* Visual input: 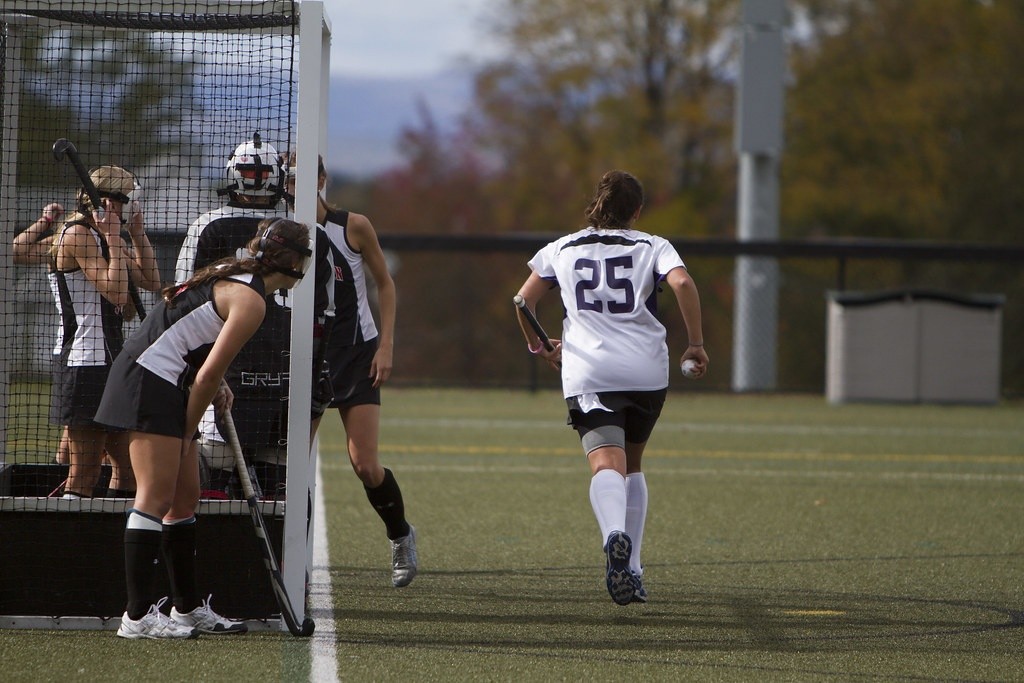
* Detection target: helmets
[226,139,284,198]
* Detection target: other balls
[680,359,701,379]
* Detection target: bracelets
[41,215,51,227]
[688,341,704,347]
[527,341,543,354]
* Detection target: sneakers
[116,596,200,640]
[607,530,647,606]
[170,594,249,634]
[390,525,417,586]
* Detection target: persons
[513,172,709,607]
[53,166,161,501]
[172,132,336,544]
[11,184,139,462]
[281,151,419,585]
[92,217,312,637]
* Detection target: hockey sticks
[511,292,564,369]
[50,135,148,320]
[215,382,317,638]
